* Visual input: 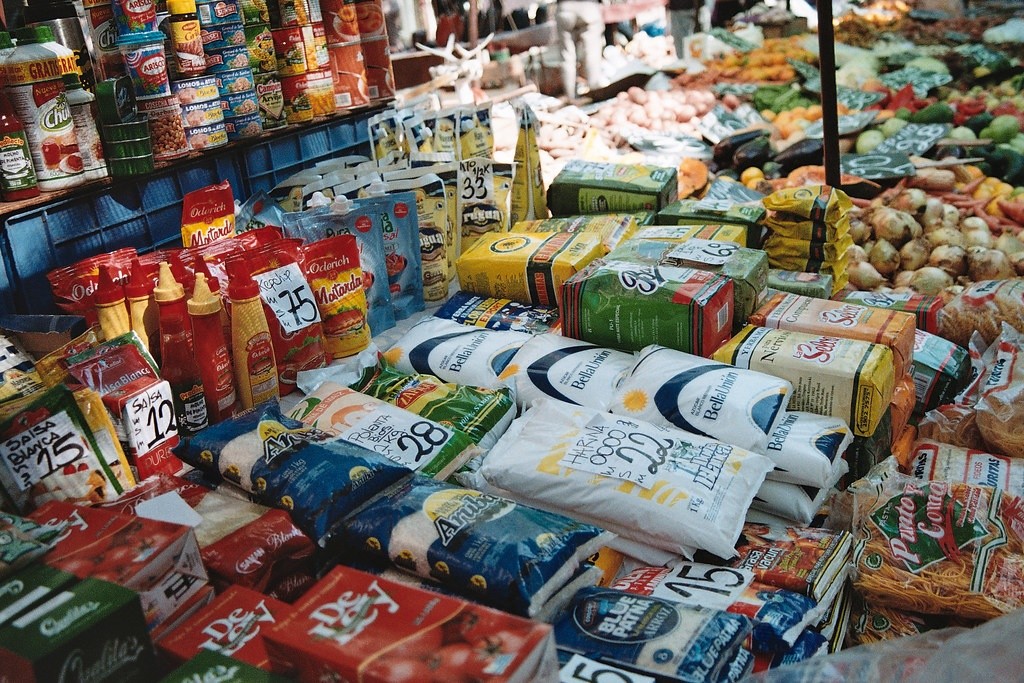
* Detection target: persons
[553,0,765,99]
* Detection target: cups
[111,0,159,34]
[115,30,171,101]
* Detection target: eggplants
[702,129,825,181]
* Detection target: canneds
[163,0,396,154]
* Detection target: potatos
[588,86,739,140]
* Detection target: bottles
[227,259,279,410]
[33,26,82,87]
[92,264,130,342]
[62,73,109,180]
[188,255,232,366]
[0,97,40,203]
[0,31,16,95]
[123,258,160,359]
[154,262,199,386]
[169,253,195,299]
[6,27,87,192]
[186,272,237,425]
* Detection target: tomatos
[375,608,531,683]
[52,519,179,586]
